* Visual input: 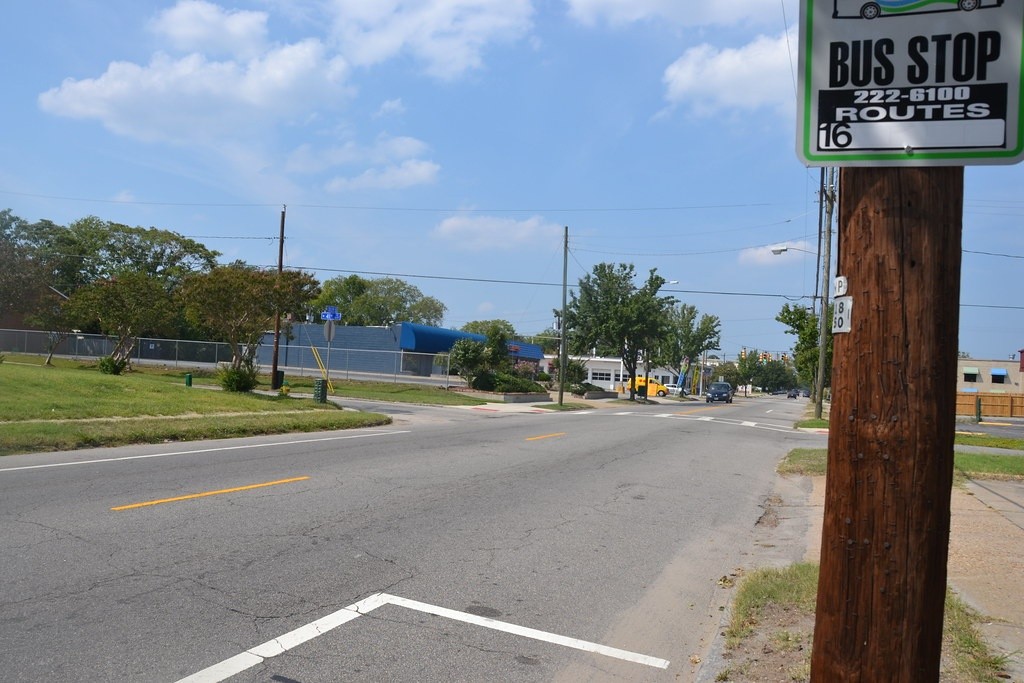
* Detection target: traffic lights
[759,352,772,363]
[741,349,747,360]
[781,353,788,363]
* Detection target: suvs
[664,384,690,396]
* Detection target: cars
[787,389,811,399]
[706,382,735,403]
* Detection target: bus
[627,377,670,397]
